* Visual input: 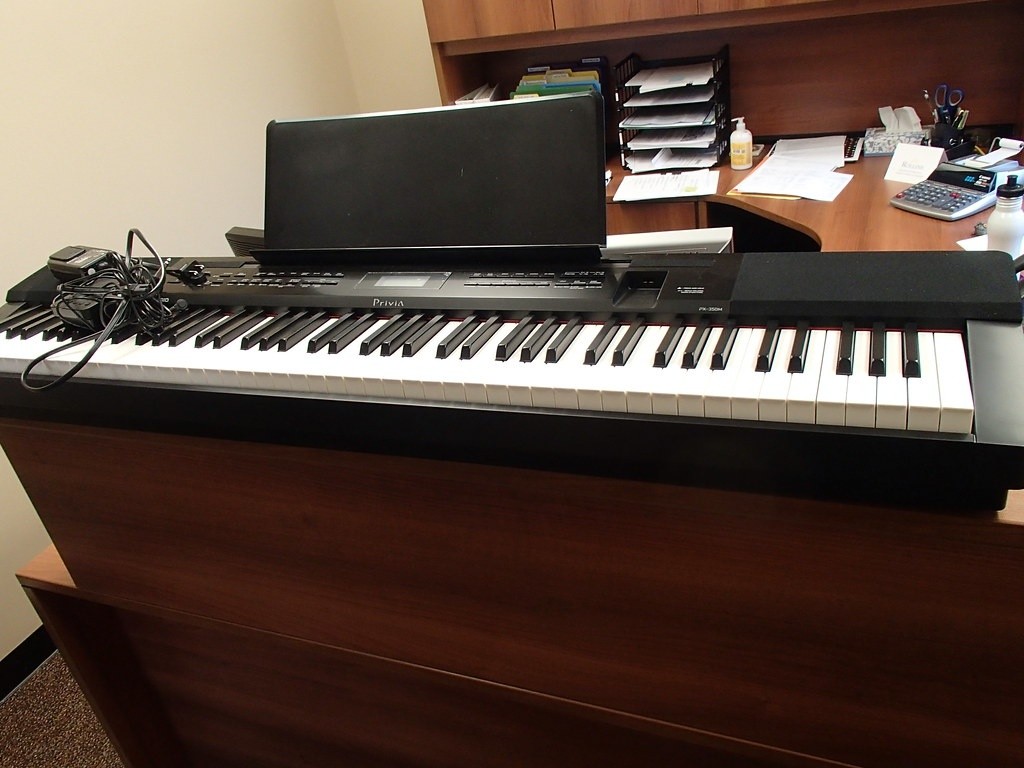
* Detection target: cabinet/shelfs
[599,196,708,230]
[424,1,1024,193]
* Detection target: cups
[937,121,965,150]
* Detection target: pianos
[0,243,1024,513]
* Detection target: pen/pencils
[930,107,969,129]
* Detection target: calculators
[890,162,998,222]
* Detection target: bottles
[730,117,753,170]
[987,175,1024,259]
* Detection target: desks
[606,148,1024,252]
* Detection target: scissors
[933,83,965,122]
